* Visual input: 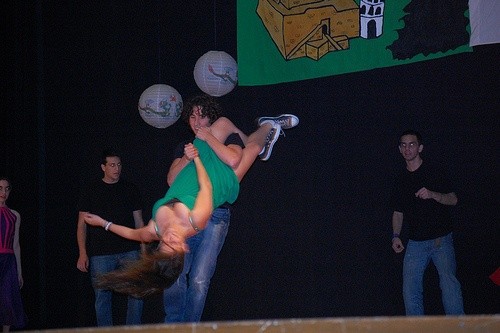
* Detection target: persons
[162,95,245,323]
[84,114,299,299]
[76,152,148,327]
[0,177,27,333]
[392,130,466,316]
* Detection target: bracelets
[105,221,112,231]
[392,234,399,241]
[439,193,442,203]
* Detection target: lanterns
[193,52,238,97]
[138,84,183,128]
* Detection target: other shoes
[257,123,281,161]
[257,113,300,129]
